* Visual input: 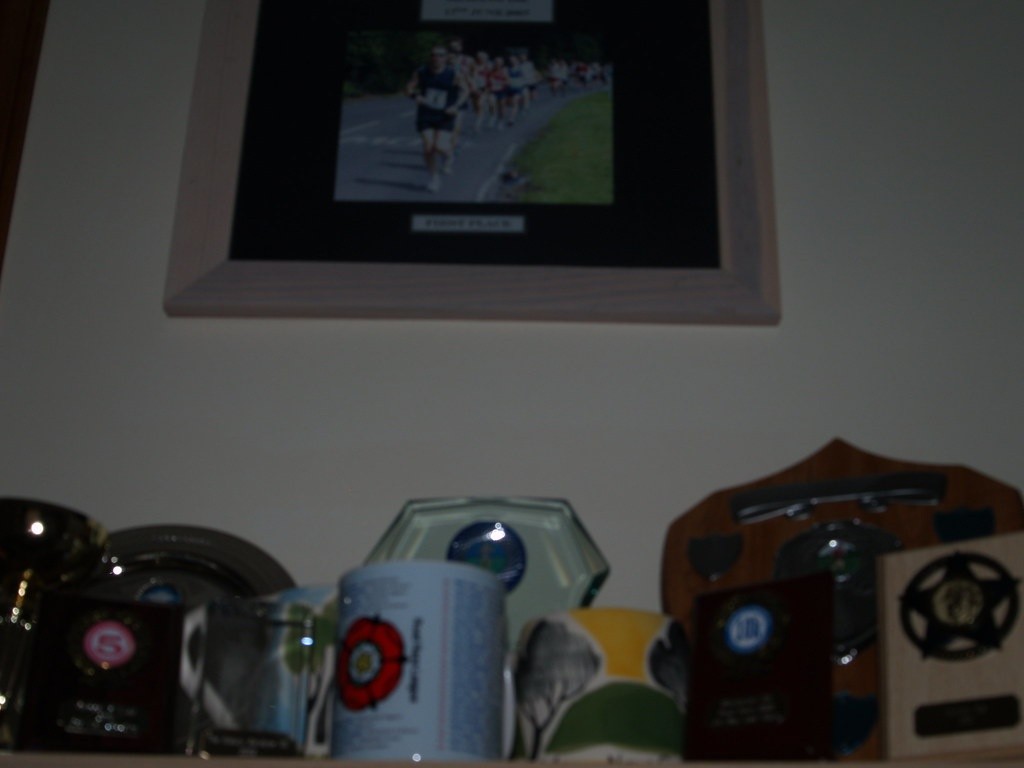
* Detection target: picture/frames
[163,1,782,326]
